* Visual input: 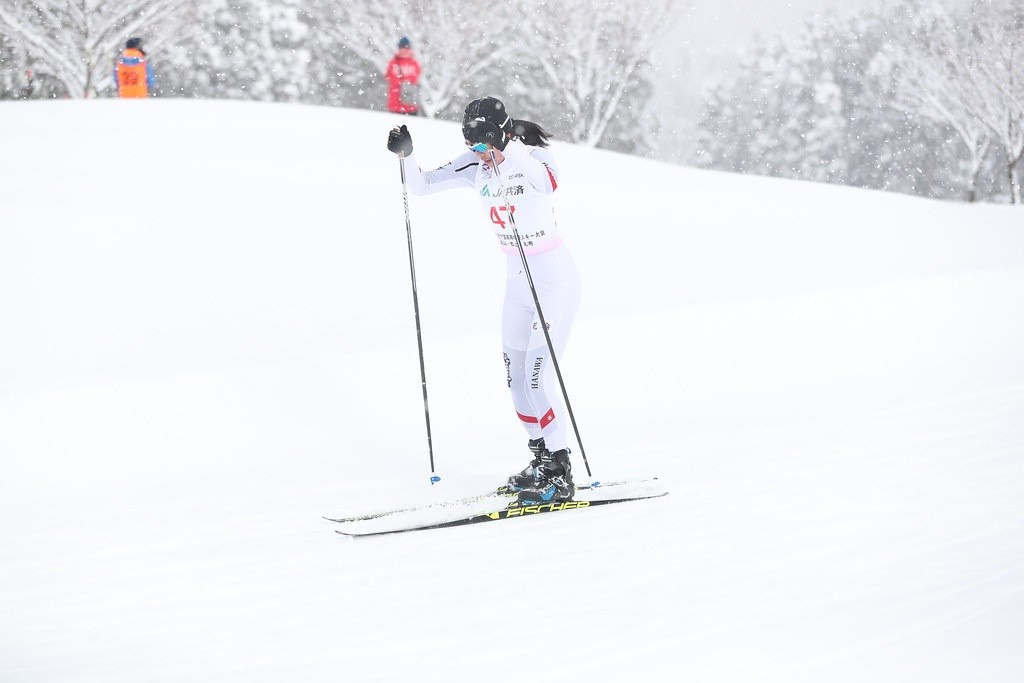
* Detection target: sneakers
[508,437,545,488]
[518,448,575,502]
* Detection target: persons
[384,37,422,115]
[113,37,155,98]
[387,96,582,505]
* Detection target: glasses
[464,141,488,152]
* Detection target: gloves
[388,125,413,157]
[462,117,509,151]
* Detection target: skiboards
[321,477,669,539]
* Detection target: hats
[126,38,140,47]
[398,37,409,48]
[461,95,511,135]
